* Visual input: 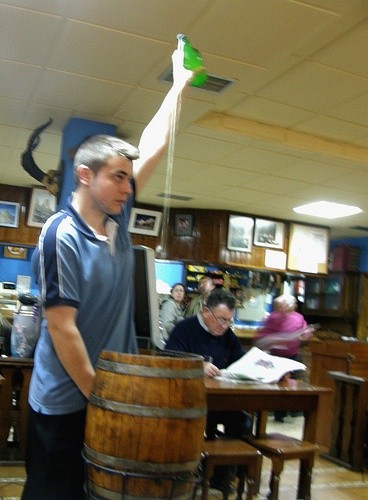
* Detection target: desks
[319,368,368,475]
[202,371,336,500]
[0,356,35,467]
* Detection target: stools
[245,433,330,500]
[195,439,263,500]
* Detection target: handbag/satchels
[10,314,41,358]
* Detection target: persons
[20,48,206,500]
[184,276,215,318]
[160,283,185,343]
[164,289,245,495]
[251,293,313,423]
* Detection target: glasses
[203,305,234,328]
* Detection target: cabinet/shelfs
[183,264,357,338]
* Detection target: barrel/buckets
[82,347,208,500]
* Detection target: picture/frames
[174,212,194,236]
[254,217,285,249]
[226,214,254,252]
[0,199,21,228]
[128,206,163,237]
[26,185,59,228]
